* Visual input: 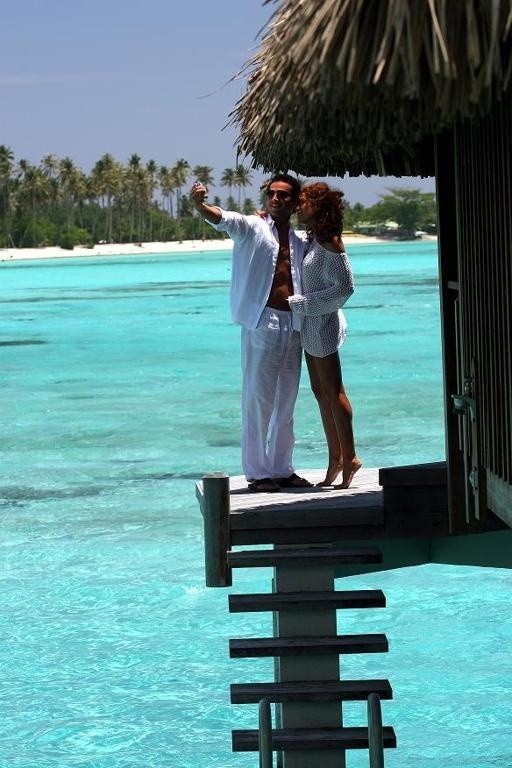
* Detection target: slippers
[248,478,280,492]
[273,473,314,488]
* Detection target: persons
[189,173,314,493]
[254,181,362,490]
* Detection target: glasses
[266,190,291,199]
